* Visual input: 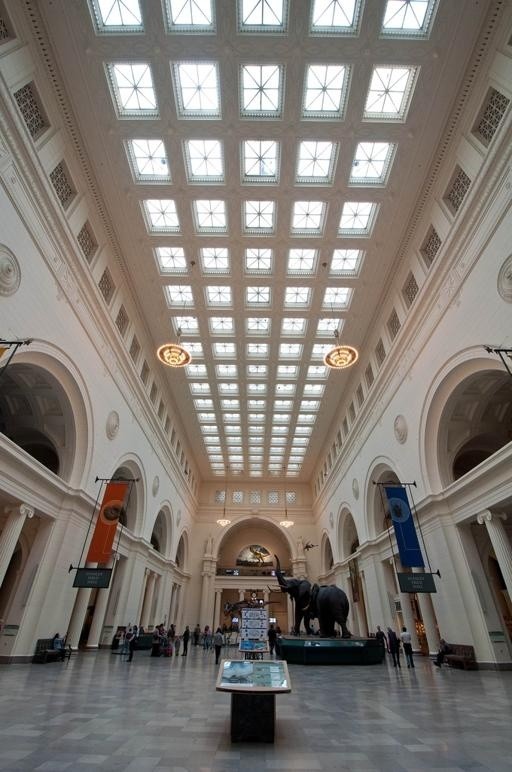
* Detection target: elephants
[266,554,351,638]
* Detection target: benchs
[442,643,474,670]
[32,637,66,664]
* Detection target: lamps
[215,464,296,529]
[321,262,359,371]
[155,260,196,369]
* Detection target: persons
[201,625,209,649]
[431,639,451,667]
[193,623,201,647]
[398,627,415,669]
[181,625,190,656]
[375,625,388,662]
[213,627,224,664]
[174,636,180,656]
[266,624,277,656]
[274,626,281,634]
[52,632,67,662]
[385,626,401,668]
[109,620,176,662]
[219,622,239,633]
